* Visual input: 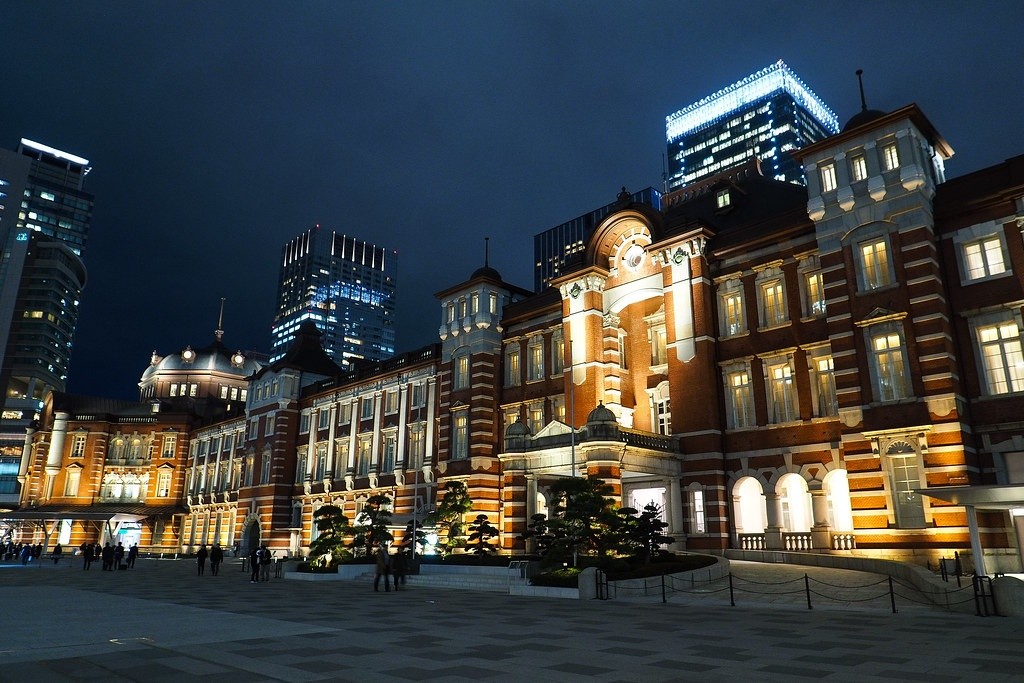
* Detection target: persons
[373,545,407,592]
[127,542,138,568]
[80,542,102,570]
[210,543,223,576]
[0,542,42,566]
[102,542,124,571]
[53,544,62,564]
[233,542,239,557]
[250,545,271,584]
[196,544,208,577]
